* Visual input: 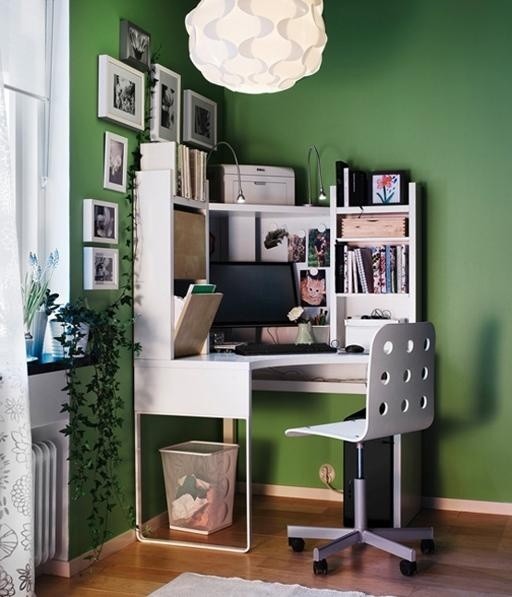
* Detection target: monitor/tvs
[208,261,302,344]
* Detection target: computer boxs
[343,407,393,528]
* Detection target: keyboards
[236,342,337,356]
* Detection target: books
[139,141,206,200]
[341,243,409,294]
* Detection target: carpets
[146,570,402,597]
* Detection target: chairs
[284,320,438,579]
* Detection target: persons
[314,233,325,266]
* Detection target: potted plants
[28,288,60,356]
[23,278,50,363]
[48,42,161,577]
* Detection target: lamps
[206,139,246,204]
[302,144,328,207]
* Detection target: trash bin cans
[158,441,240,535]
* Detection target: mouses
[345,344,365,354]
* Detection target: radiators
[28,438,57,567]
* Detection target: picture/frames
[367,167,411,204]
[82,16,219,293]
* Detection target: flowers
[23,247,60,335]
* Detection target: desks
[133,167,424,554]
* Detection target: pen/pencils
[309,309,328,325]
